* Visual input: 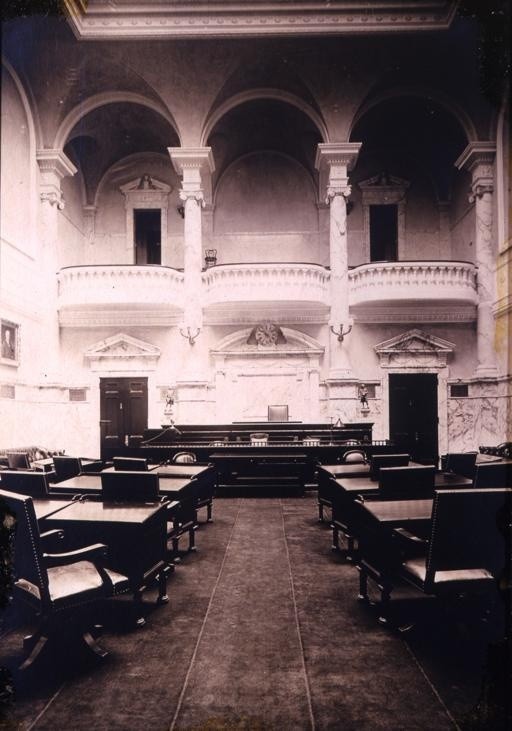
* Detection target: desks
[1,447,217,625]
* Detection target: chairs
[208,432,388,446]
[316,442,512,637]
[1,489,130,673]
